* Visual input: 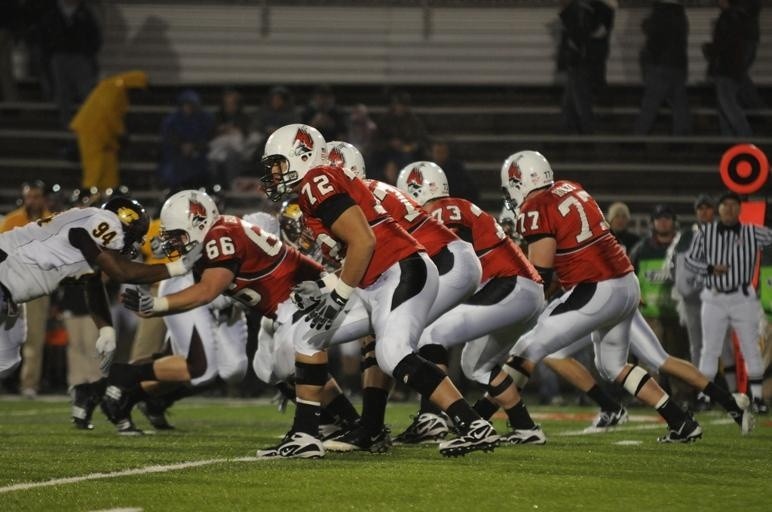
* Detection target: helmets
[650,204,676,221]
[496,150,554,212]
[101,190,221,263]
[259,124,450,258]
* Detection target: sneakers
[583,393,756,443]
[256,409,547,460]
[67,358,175,436]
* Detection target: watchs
[620,0,698,153]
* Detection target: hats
[693,191,741,209]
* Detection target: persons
[702,2,758,138]
[255,122,502,461]
[66,66,152,197]
[1,0,97,110]
[143,81,482,209]
[1,139,770,453]
[550,2,614,165]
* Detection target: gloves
[165,242,205,277]
[92,326,117,373]
[288,278,349,331]
[121,286,169,318]
